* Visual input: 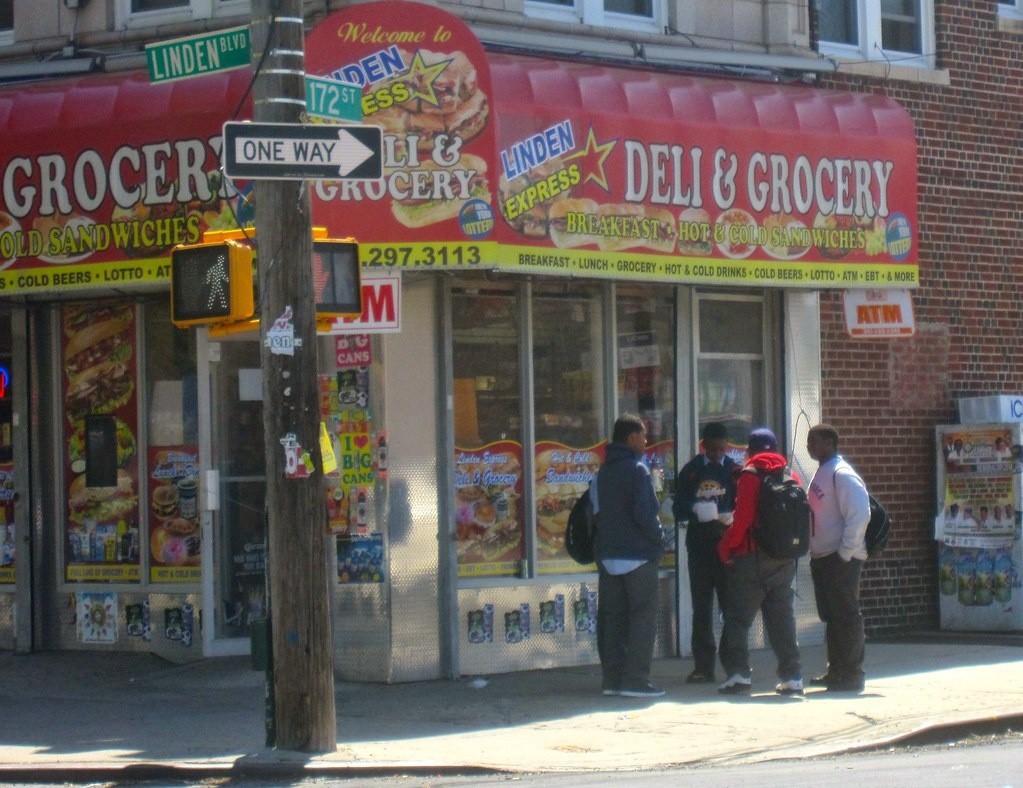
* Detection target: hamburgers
[457,450,522,564]
[359,49,490,226]
[534,450,596,559]
[64,301,138,527]
[501,155,712,257]
[151,483,195,535]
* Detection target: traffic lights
[169,237,255,330]
[308,236,365,322]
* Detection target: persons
[807,424,873,693]
[670,421,746,684]
[718,429,806,698]
[582,411,670,698]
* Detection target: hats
[747,428,777,450]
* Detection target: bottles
[116,513,127,562]
[957,549,975,606]
[976,548,994,606]
[994,547,1012,603]
[940,544,957,596]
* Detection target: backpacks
[563,476,600,569]
[742,465,816,558]
[833,466,895,557]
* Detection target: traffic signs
[143,23,255,87]
[304,71,366,123]
[221,118,386,183]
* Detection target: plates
[758,223,811,259]
[715,209,759,259]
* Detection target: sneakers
[599,685,664,696]
[685,667,716,684]
[774,679,804,694]
[810,687,866,697]
[811,672,830,684]
[718,671,750,693]
